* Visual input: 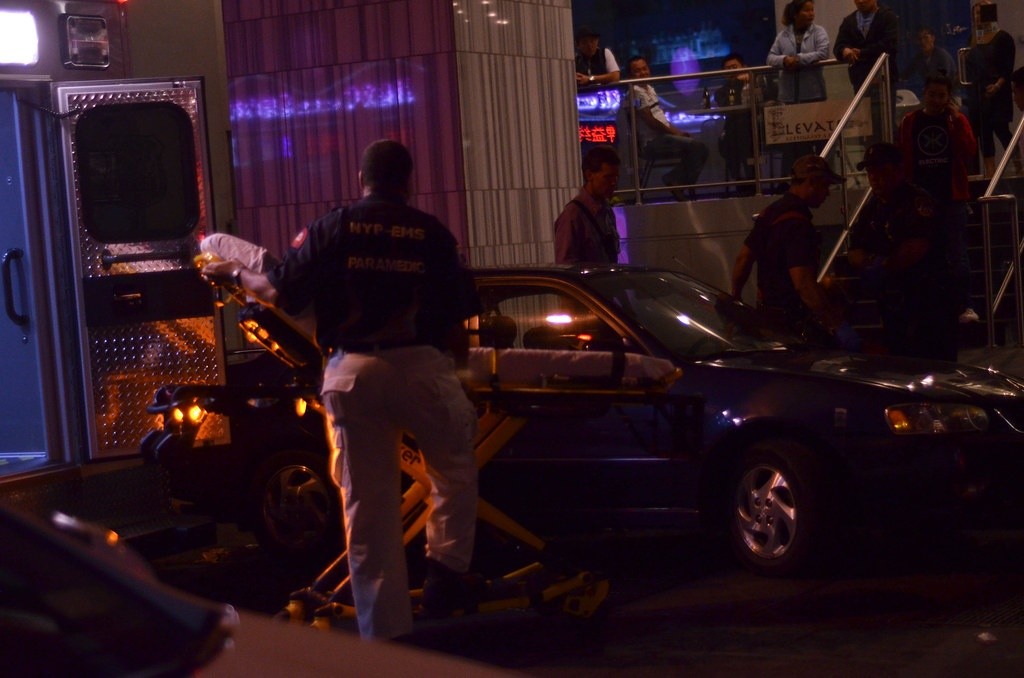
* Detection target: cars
[0,483,500,678]
[228,261,1024,578]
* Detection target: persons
[840,143,958,360]
[575,0,1024,203]
[553,147,620,324]
[202,139,480,644]
[895,72,979,322]
[729,155,858,351]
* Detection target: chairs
[624,103,696,204]
[522,325,563,348]
[478,315,517,349]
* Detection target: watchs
[232,266,245,287]
[589,75,595,83]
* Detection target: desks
[681,99,776,189]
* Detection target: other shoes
[663,172,690,202]
[421,567,490,607]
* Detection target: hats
[856,143,903,172]
[792,156,846,186]
[576,26,601,40]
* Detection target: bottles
[701,87,711,108]
[743,82,751,107]
[627,20,729,65]
[728,83,735,105]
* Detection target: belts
[345,341,420,354]
